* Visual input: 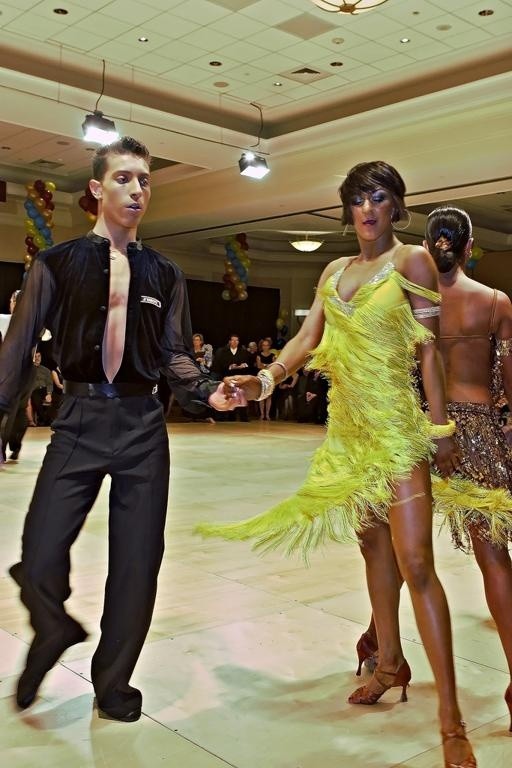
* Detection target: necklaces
[107,247,128,260]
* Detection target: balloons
[220,233,252,302]
[21,180,57,288]
[79,186,97,225]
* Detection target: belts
[63,380,160,399]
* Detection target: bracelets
[259,367,276,396]
[265,362,289,386]
[427,418,457,442]
[258,373,272,403]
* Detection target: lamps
[235,101,270,181]
[311,1,389,16]
[79,57,119,144]
[284,231,324,252]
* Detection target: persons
[0,136,250,721]
[295,369,329,424]
[25,352,63,427]
[222,161,481,768]
[193,334,299,424]
[338,203,512,730]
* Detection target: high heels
[357,616,380,671]
[348,660,413,706]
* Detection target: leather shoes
[12,617,86,711]
[438,718,477,767]
[93,686,145,720]
